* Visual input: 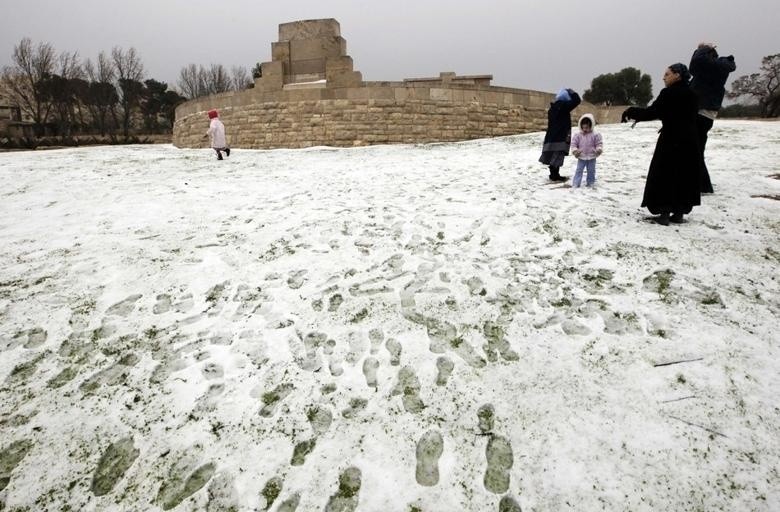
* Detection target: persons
[620,61,713,225]
[203,109,231,161]
[688,42,736,194]
[570,113,603,188]
[538,88,582,181]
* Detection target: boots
[217,148,230,160]
[549,165,714,224]
